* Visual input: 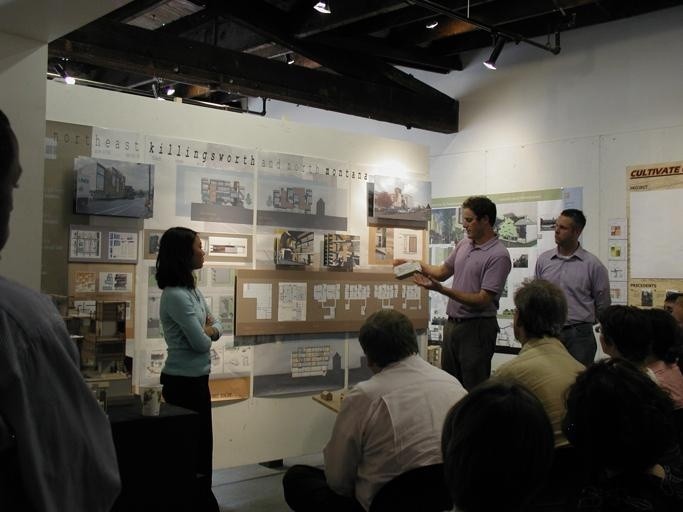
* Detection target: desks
[106,393,198,488]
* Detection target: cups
[139,385,164,415]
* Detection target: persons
[598,293,683,411]
[282,309,474,511]
[154,227,223,512]
[371,357,682,512]
[496,279,588,458]
[0,108,126,512]
[541,209,609,365]
[392,193,512,392]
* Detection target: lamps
[53,61,76,86]
[481,31,508,73]
